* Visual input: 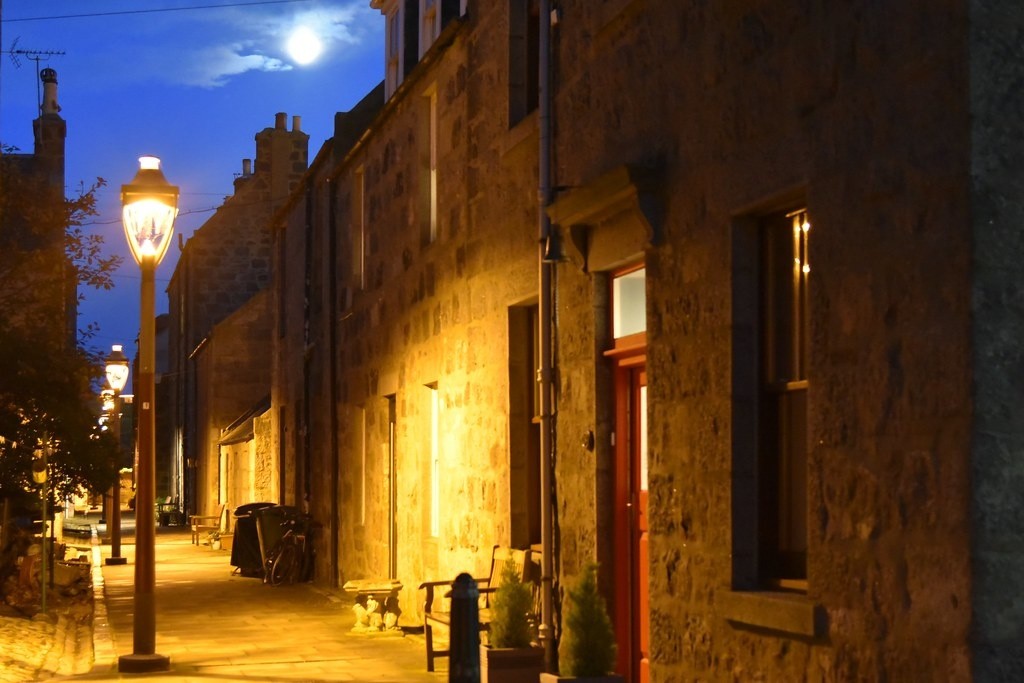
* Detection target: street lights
[118,153,181,669]
[96,344,135,565]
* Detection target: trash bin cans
[254,505,298,585]
[231,503,275,578]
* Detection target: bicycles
[264,505,312,589]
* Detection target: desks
[345,580,406,637]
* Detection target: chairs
[189,504,225,546]
[158,494,179,527]
[419,545,532,672]
